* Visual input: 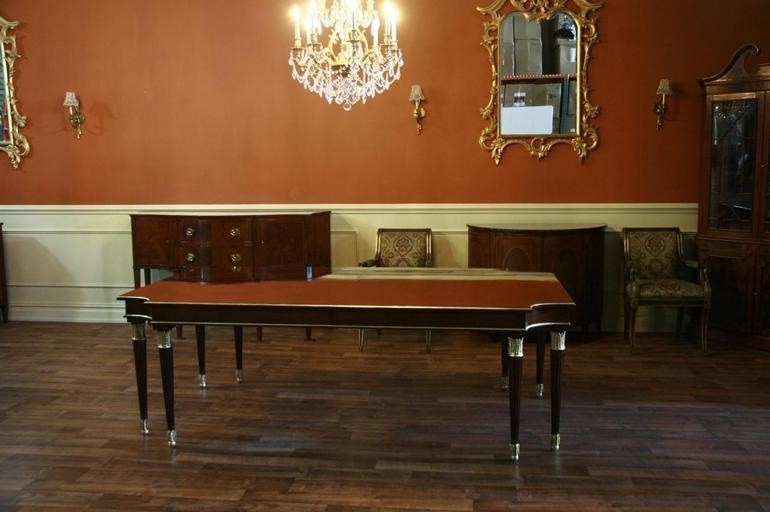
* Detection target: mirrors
[710,98,759,231]
[474,1,602,166]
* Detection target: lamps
[61,91,87,138]
[408,84,429,136]
[285,0,404,113]
[651,78,675,132]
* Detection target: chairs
[357,227,435,353]
[621,226,714,356]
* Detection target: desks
[464,222,610,343]
[116,266,576,463]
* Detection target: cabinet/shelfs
[697,41,770,354]
[129,210,333,341]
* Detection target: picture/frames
[0,14,34,170]
